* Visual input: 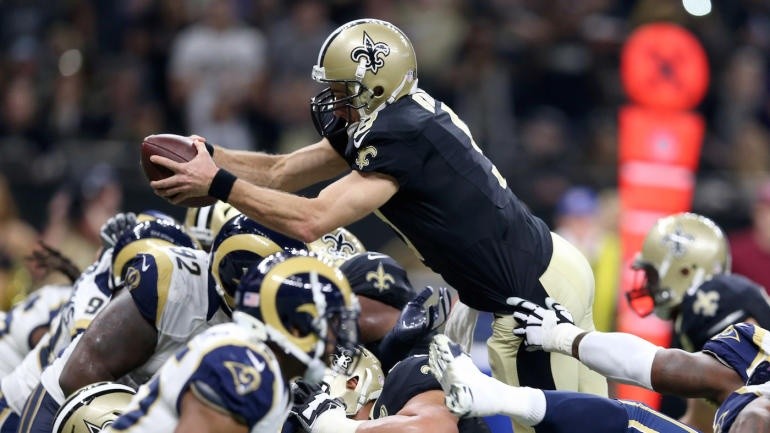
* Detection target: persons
[0,0,770,342]
[428,212,770,433]
[0,198,490,433]
[148,19,609,433]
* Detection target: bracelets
[571,331,588,360]
[208,169,237,203]
[205,142,214,157]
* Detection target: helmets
[206,201,244,235]
[50,380,136,433]
[112,221,198,280]
[208,213,308,316]
[625,213,732,324]
[309,19,417,137]
[232,248,361,380]
[302,227,367,269]
[326,344,385,416]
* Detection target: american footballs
[140,133,218,208]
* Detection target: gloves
[507,296,585,356]
[100,213,138,247]
[289,378,367,433]
[390,286,452,349]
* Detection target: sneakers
[430,334,486,419]
